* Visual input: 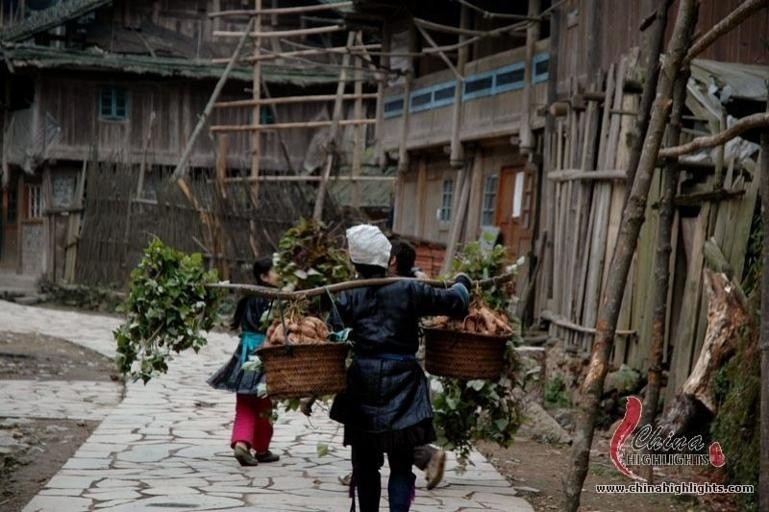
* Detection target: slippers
[338,472,352,486]
[424,449,446,490]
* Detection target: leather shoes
[235,446,258,466]
[255,452,280,462]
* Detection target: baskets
[423,327,510,380]
[251,341,351,397]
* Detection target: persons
[336,236,447,491]
[208,255,281,467]
[296,221,474,511]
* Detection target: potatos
[263,315,330,349]
[426,303,512,334]
